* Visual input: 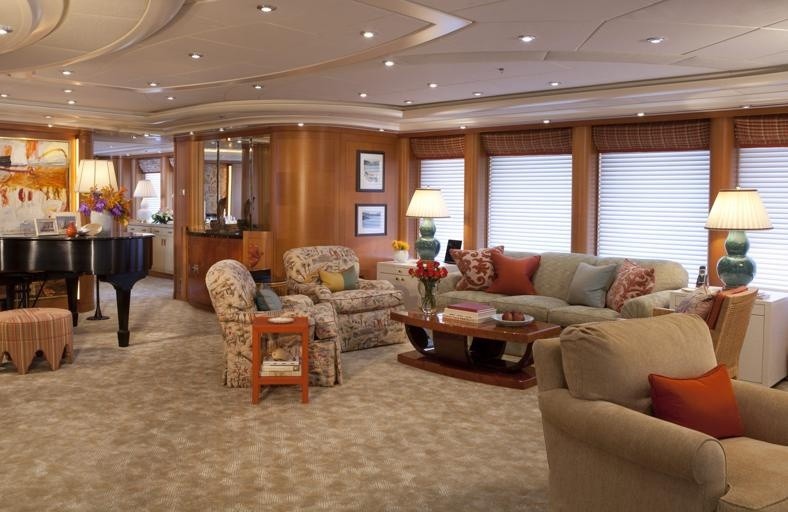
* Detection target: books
[443,302,496,320]
[260,356,301,377]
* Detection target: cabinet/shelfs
[669,285,788,387]
[376,259,459,309]
[127,223,174,275]
[187,229,242,313]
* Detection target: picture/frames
[34,218,59,236]
[51,212,81,234]
[0,137,72,301]
[355,203,387,237]
[444,240,462,264]
[204,164,229,217]
[355,149,385,192]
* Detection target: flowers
[151,208,174,221]
[408,260,448,311]
[390,240,410,250]
[78,183,133,226]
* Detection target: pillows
[341,264,360,289]
[314,301,339,339]
[605,258,655,313]
[648,362,745,438]
[566,261,618,308]
[255,281,282,310]
[318,265,344,292]
[485,251,541,296]
[676,283,713,321]
[449,246,505,291]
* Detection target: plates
[491,313,534,326]
[268,317,294,323]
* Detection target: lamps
[73,159,119,193]
[405,185,452,263]
[704,187,774,288]
[133,180,158,220]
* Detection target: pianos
[0,230,156,347]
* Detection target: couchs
[653,287,760,379]
[435,250,689,327]
[532,312,788,512]
[283,245,409,352]
[205,258,343,388]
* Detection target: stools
[0,307,75,375]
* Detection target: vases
[417,280,439,313]
[394,249,408,263]
[90,210,114,232]
[158,219,168,224]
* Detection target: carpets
[0,296,550,511]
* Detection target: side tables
[252,316,309,404]
